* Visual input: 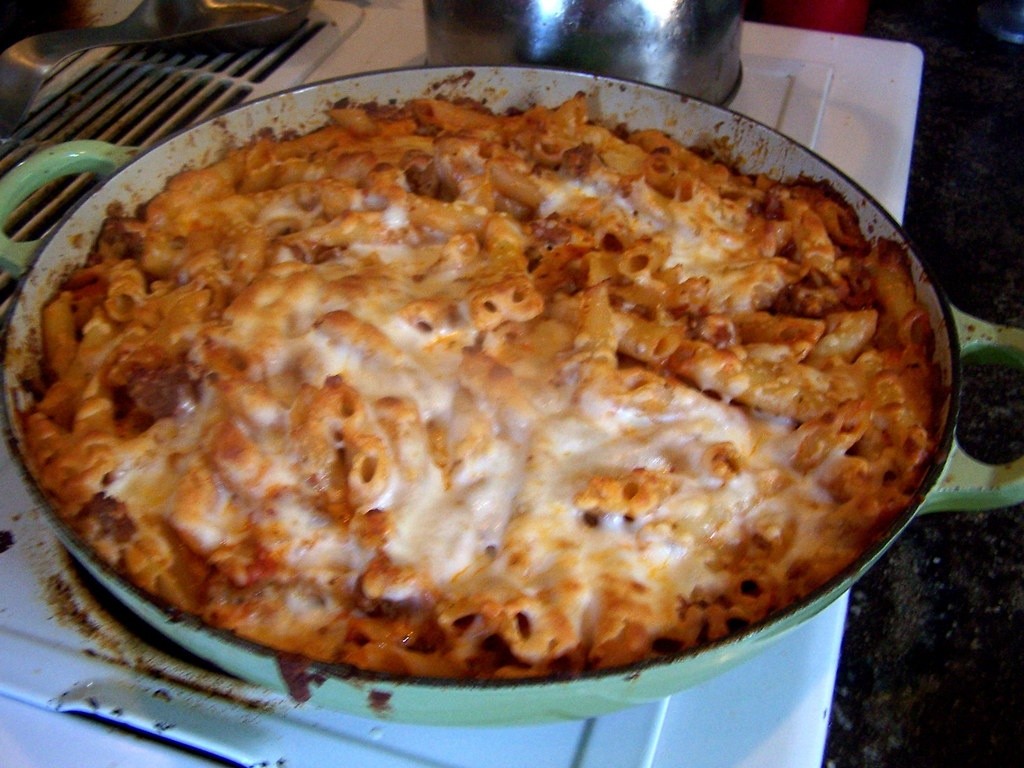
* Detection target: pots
[0,65,1024,729]
